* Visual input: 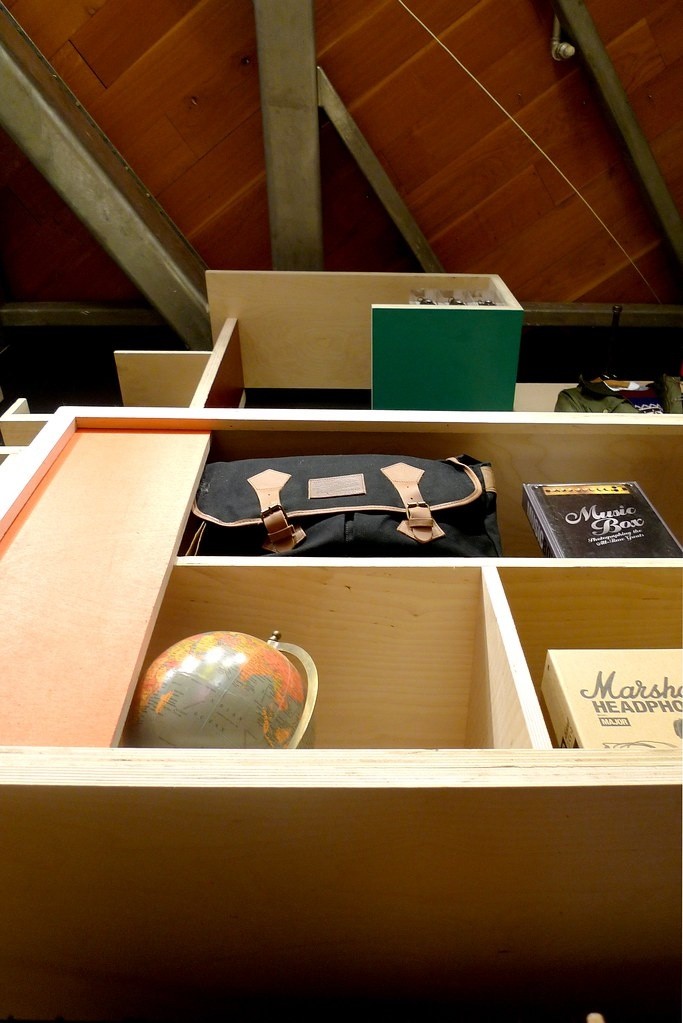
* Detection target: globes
[126,631,318,748]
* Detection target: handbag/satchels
[184,453,504,557]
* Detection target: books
[522,481,683,558]
[540,649,682,749]
